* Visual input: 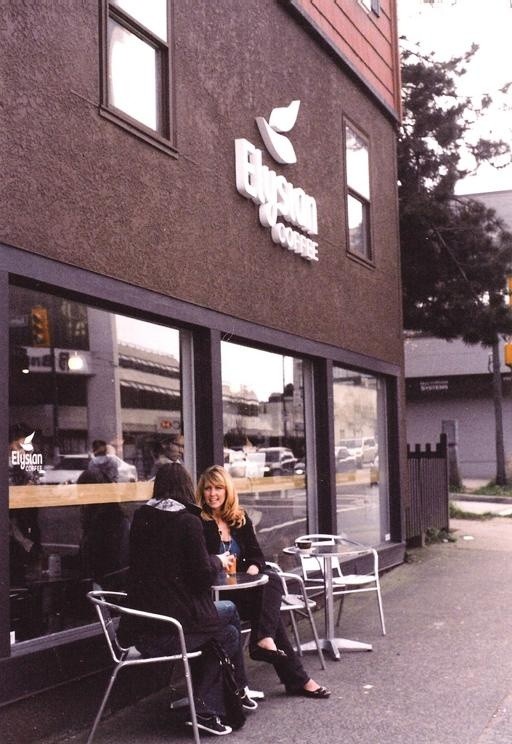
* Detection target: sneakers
[239,690,258,710]
[184,713,233,736]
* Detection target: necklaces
[222,532,232,553]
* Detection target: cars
[221,437,380,477]
[38,455,139,488]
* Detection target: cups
[47,553,62,577]
[223,552,237,576]
[224,576,238,585]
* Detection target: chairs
[261,561,330,671]
[295,532,386,638]
[86,589,206,744]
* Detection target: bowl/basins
[295,541,311,549]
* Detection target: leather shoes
[249,643,288,663]
[297,686,331,698]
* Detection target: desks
[169,570,267,714]
[282,545,374,663]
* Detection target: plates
[294,548,317,552]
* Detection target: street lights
[50,339,86,456]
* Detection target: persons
[89,440,118,483]
[195,464,332,698]
[9,434,40,589]
[148,444,173,481]
[116,462,258,735]
[41,469,131,635]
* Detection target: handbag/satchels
[219,658,246,729]
